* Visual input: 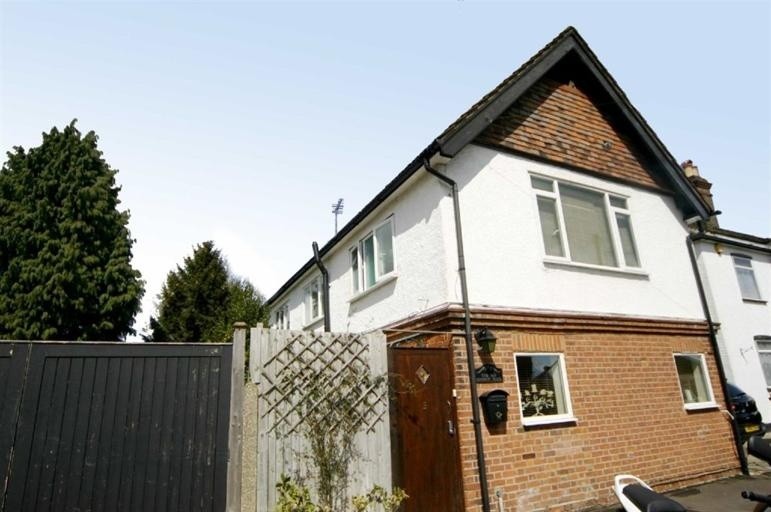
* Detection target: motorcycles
[606,473,688,512]
[734,432,771,506]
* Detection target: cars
[728,383,767,440]
